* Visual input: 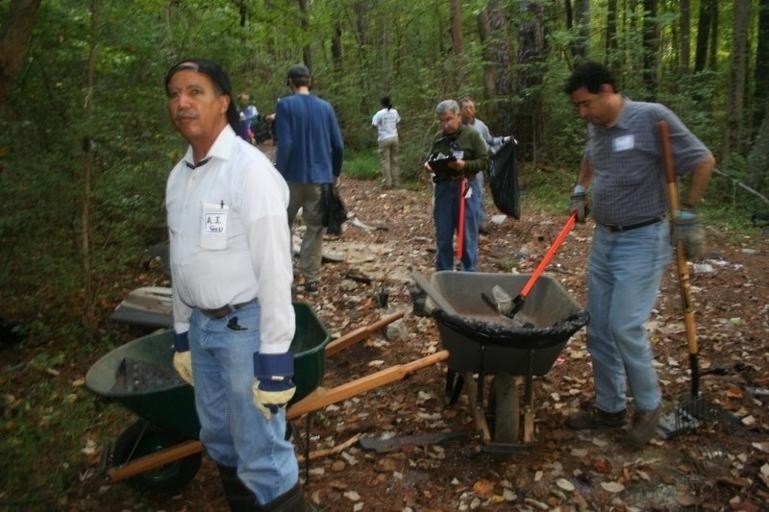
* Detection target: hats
[286,65,312,87]
[163,56,241,137]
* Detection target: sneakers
[303,276,323,296]
[622,401,663,452]
[562,404,628,430]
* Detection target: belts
[599,212,666,235]
[185,295,266,320]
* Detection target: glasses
[437,115,459,125]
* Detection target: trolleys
[406,265,592,455]
[79,299,451,491]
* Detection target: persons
[457,96,519,234]
[423,100,490,272]
[274,65,344,296]
[371,96,401,189]
[236,94,259,145]
[563,59,716,451]
[264,113,276,145]
[165,60,306,512]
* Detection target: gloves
[502,132,521,148]
[249,348,298,421]
[667,209,706,261]
[566,190,592,226]
[169,329,201,388]
